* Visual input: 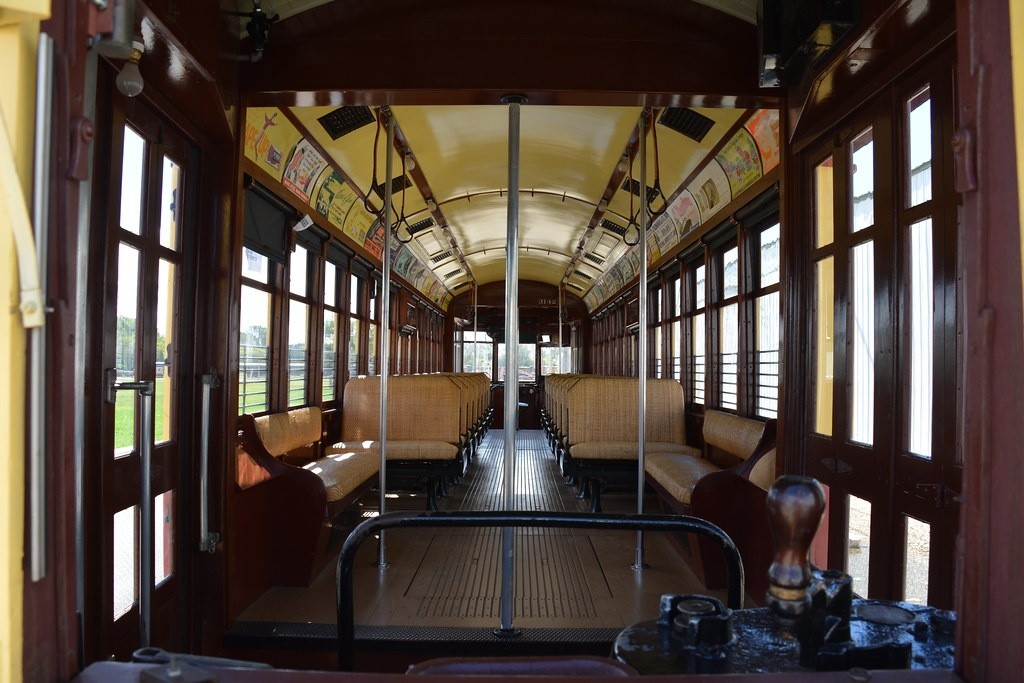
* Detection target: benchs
[237,372,495,587]
[542,373,776,591]
